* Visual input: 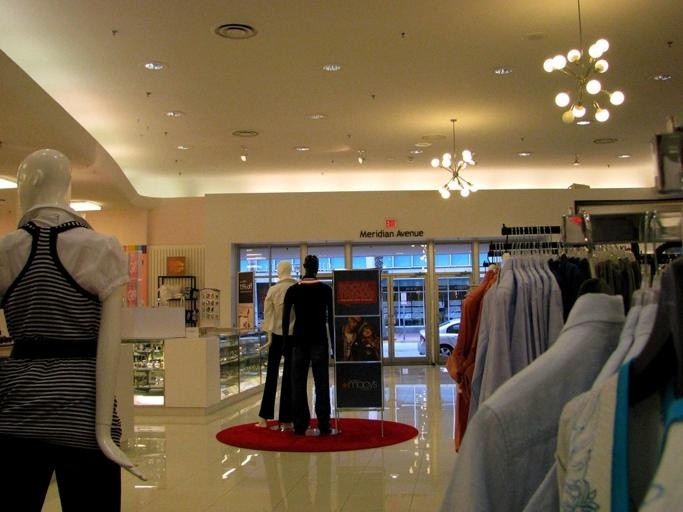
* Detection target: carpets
[216,418,419,452]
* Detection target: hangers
[488,211,663,306]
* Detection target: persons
[255,258,296,428]
[338,316,380,361]
[281,255,334,435]
[0,148,146,511]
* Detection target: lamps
[430,119,479,200]
[543,1,625,124]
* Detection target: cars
[418,318,461,357]
